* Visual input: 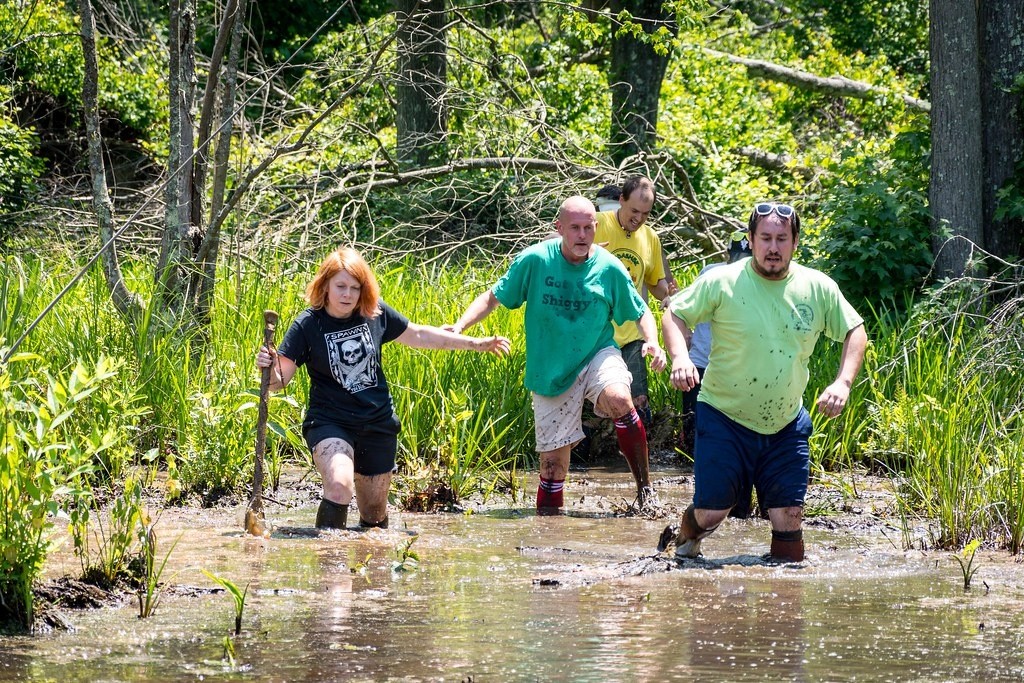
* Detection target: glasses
[752,203,796,226]
[731,231,749,242]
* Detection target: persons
[660,231,753,473]
[439,196,667,515]
[254,248,510,530]
[594,175,679,431]
[661,202,867,563]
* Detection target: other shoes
[658,523,680,554]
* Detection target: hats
[727,228,752,263]
